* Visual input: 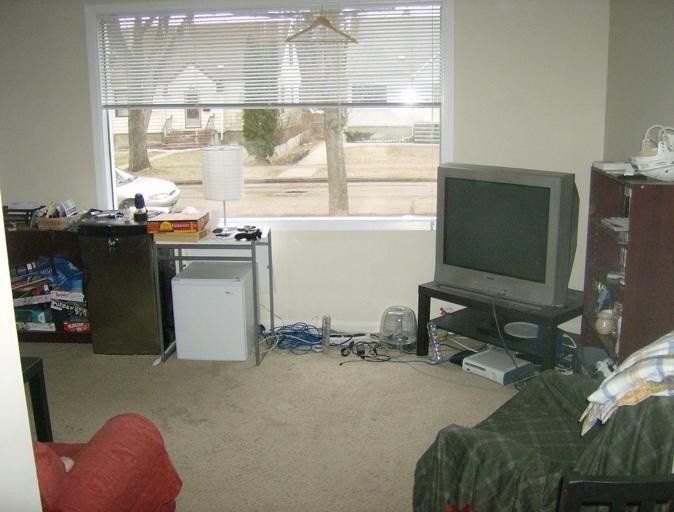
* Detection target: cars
[113,166,181,210]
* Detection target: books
[619,184,631,216]
[613,245,628,277]
[600,217,628,233]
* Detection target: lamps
[203,144,246,231]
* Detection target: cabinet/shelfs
[2,212,92,343]
[580,164,674,373]
[415,280,585,379]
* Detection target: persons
[122,201,130,222]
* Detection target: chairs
[33,411,180,511]
[412,373,674,511]
[563,474,674,510]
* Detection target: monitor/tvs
[434,162,581,312]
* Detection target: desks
[148,230,277,364]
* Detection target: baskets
[35,212,80,231]
